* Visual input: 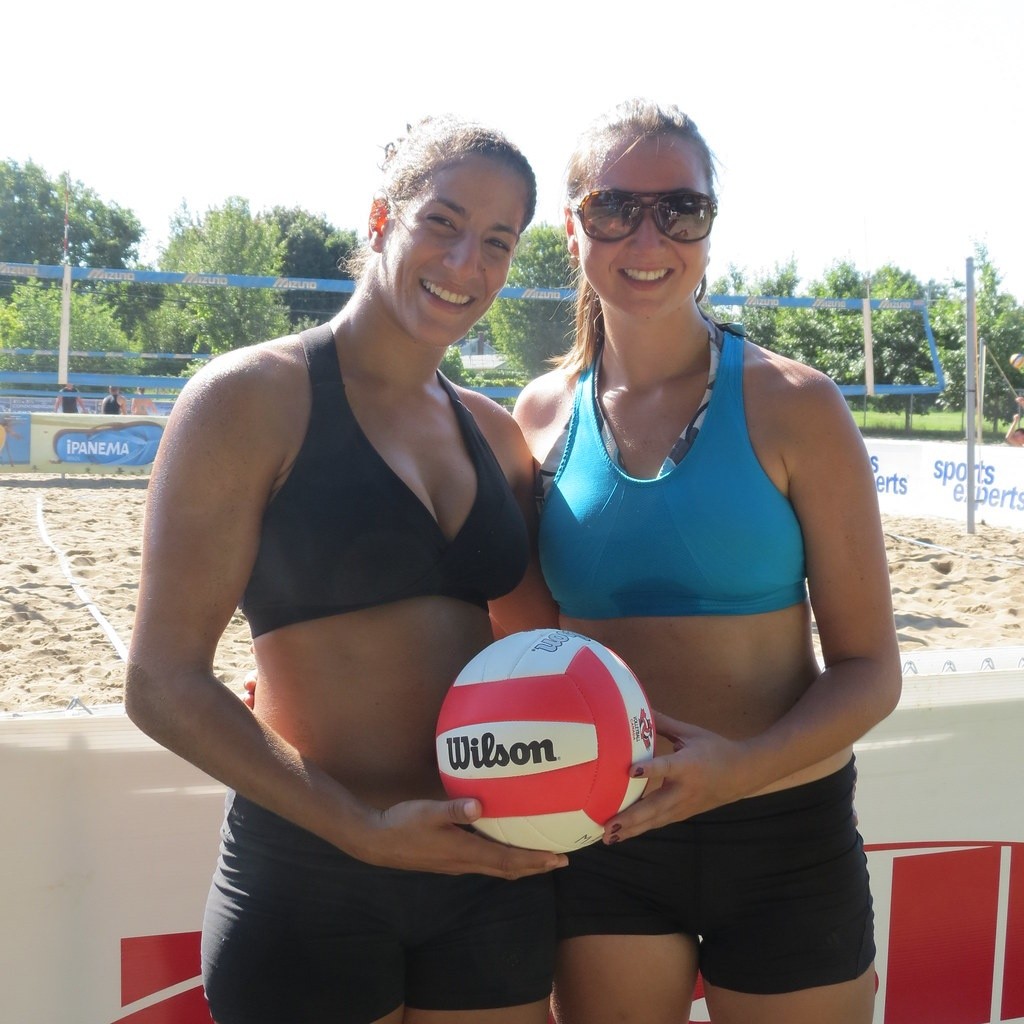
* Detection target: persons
[487,102,902,1024]
[123,116,571,1024]
[1006,397,1024,447]
[130,387,158,415]
[54,383,89,414]
[101,386,127,415]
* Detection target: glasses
[570,190,718,243]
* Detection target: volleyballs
[1009,353,1024,369]
[434,627,655,855]
